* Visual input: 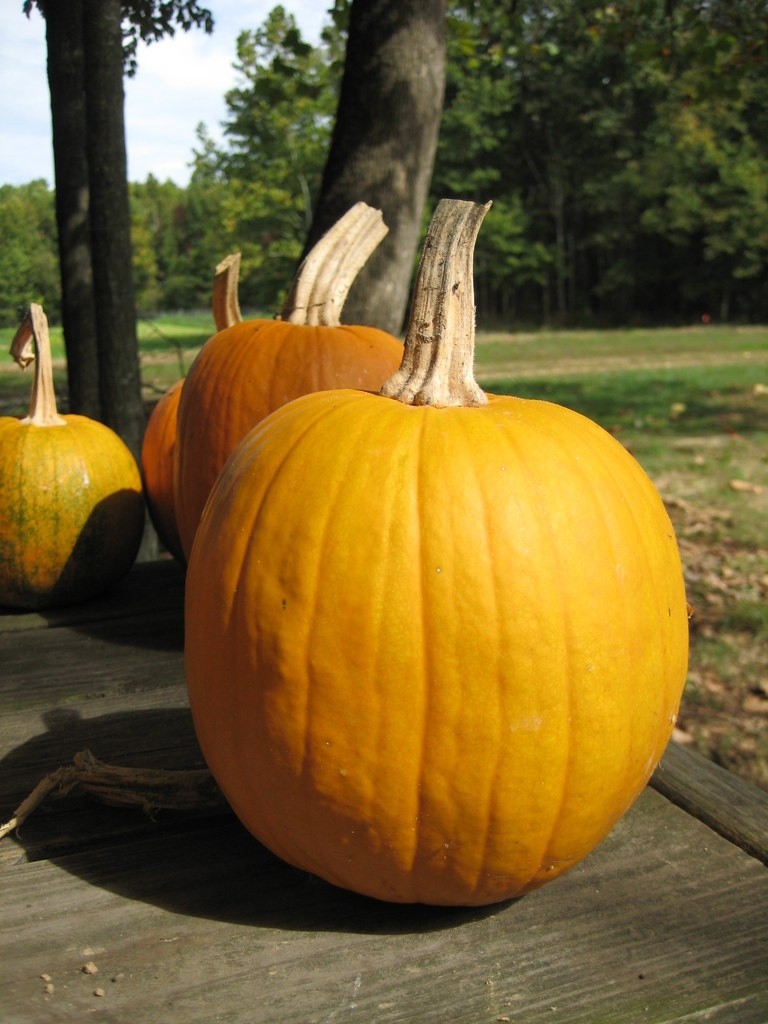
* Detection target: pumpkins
[185,200,688,904]
[0,303,145,603]
[139,201,405,574]
